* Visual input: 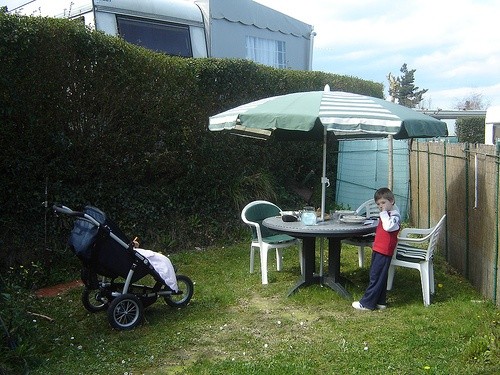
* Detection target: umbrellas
[209,85,449,277]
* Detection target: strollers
[42,201,194,331]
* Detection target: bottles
[301,206,317,225]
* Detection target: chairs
[241,198,308,285]
[384,213,448,308]
[341,199,400,269]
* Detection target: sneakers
[377,303,386,308]
[352,301,371,310]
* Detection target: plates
[342,215,366,220]
[317,213,331,221]
[335,210,356,215]
[340,218,365,223]
[279,211,300,215]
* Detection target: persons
[352,188,401,310]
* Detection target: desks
[262,210,381,302]
